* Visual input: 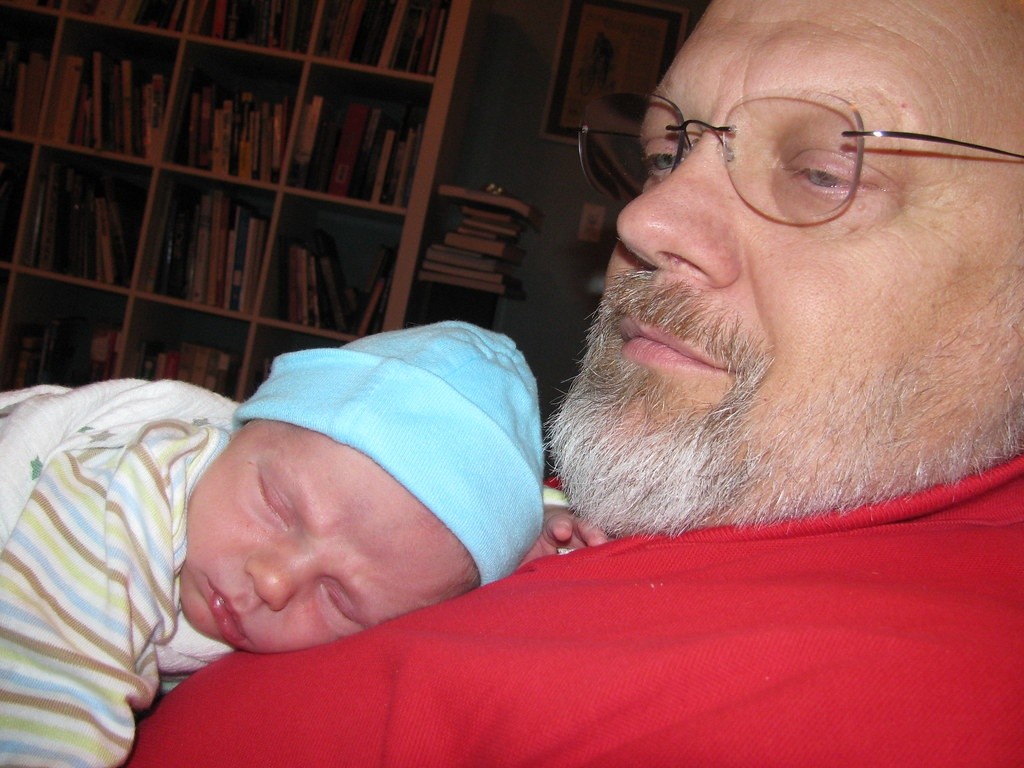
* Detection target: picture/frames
[536,1,691,147]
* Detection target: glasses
[567,85,1023,227]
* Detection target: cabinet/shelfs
[0,0,473,417]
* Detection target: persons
[0,313,607,765]
[124,2,1024,766]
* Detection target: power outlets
[577,203,605,244]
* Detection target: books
[2,3,538,396]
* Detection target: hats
[232,319,546,589]
